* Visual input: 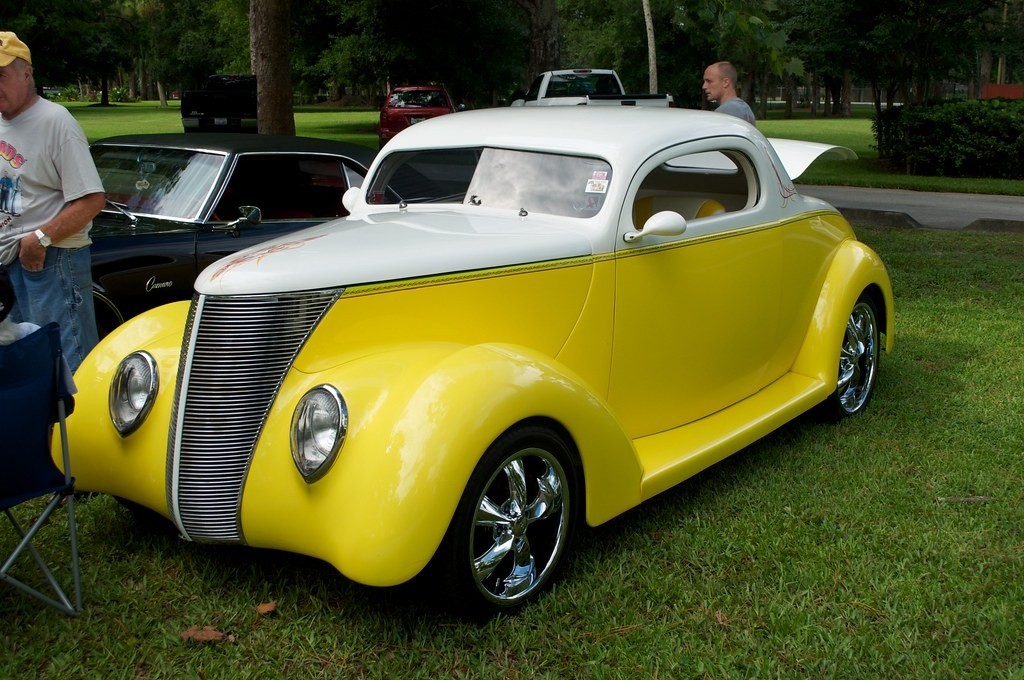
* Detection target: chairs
[0,322,84,618]
[632,192,728,233]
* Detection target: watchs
[35,229,53,249]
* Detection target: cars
[376,85,468,150]
[50,106,895,620]
[86,133,473,347]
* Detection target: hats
[0,31,32,67]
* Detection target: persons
[0,30,106,503]
[703,62,756,127]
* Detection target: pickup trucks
[181,74,257,133]
[510,68,675,109]
